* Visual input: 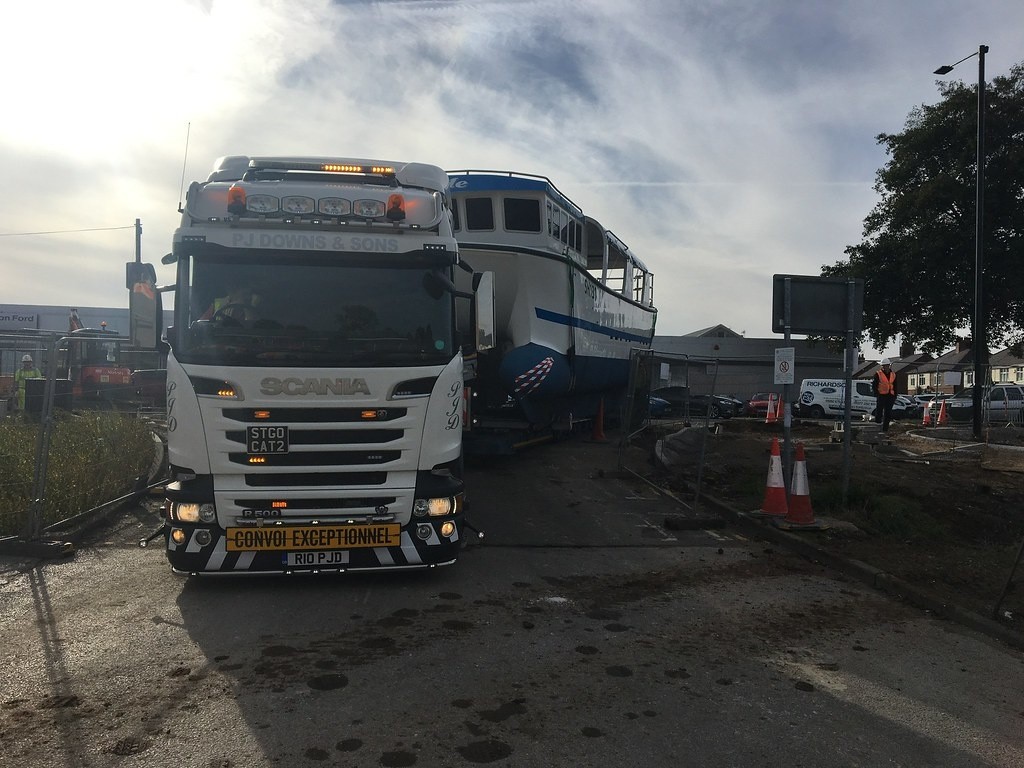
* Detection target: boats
[437,168,657,399]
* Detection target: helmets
[880,358,890,366]
[22,355,32,362]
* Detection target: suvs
[945,380,1024,425]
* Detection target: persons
[202,269,275,329]
[14,354,41,411]
[872,358,896,432]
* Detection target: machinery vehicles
[57,309,141,412]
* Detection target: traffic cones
[936,399,948,426]
[774,393,784,423]
[921,403,932,427]
[772,441,830,532]
[751,435,789,520]
[764,392,777,424]
[579,395,613,444]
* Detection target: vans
[798,378,919,420]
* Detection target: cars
[746,392,780,419]
[901,392,955,418]
[647,385,745,420]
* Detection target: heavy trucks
[127,155,657,577]
